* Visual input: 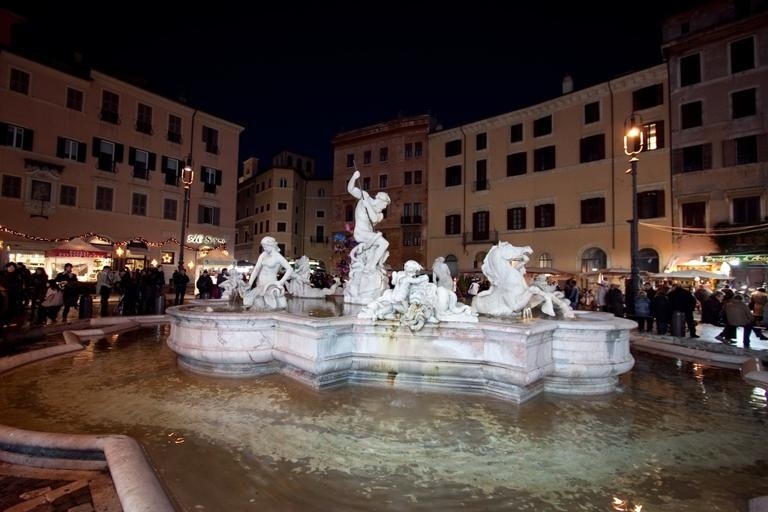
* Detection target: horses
[470,240,575,317]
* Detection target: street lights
[177,154,199,275]
[618,106,653,328]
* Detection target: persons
[390,260,429,313]
[346,171,391,273]
[565,277,768,351]
[242,236,294,309]
[454,274,490,300]
[0,259,229,329]
[432,256,454,291]
[295,255,311,294]
[309,272,345,290]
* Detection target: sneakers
[689,333,700,338]
[715,335,768,349]
